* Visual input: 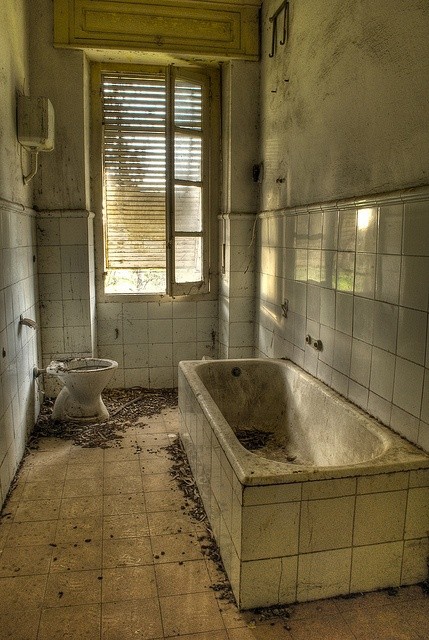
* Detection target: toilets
[48,357,119,423]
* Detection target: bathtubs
[179,358,428,611]
